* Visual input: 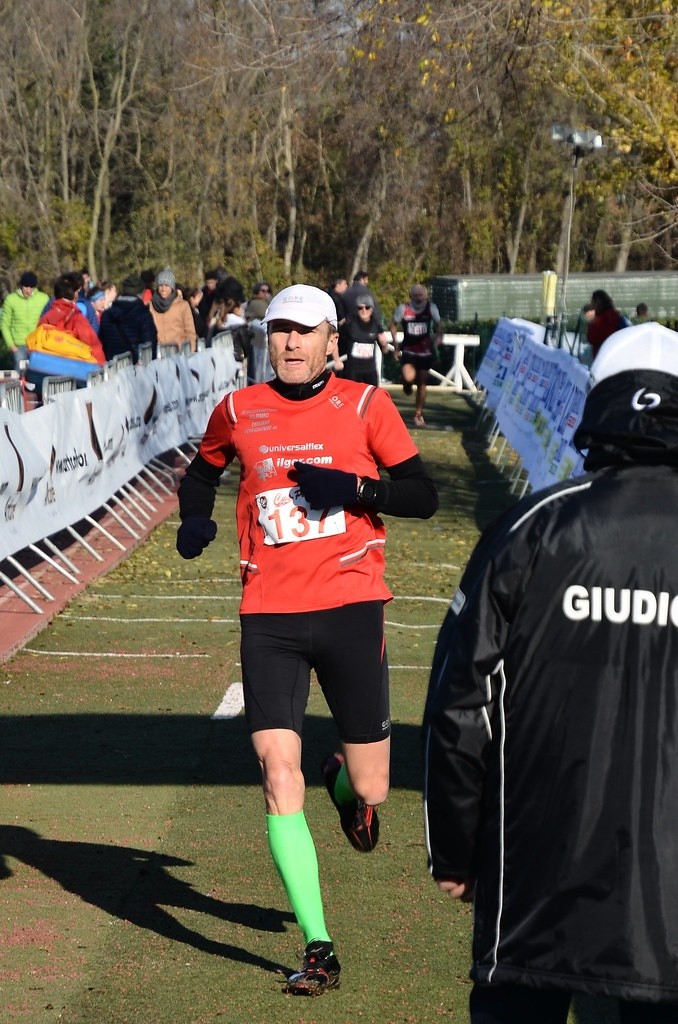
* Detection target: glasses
[357,305,371,310]
[261,289,270,294]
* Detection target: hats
[122,275,145,295]
[157,267,175,292]
[572,368,678,453]
[205,271,218,281]
[260,283,338,332]
[20,272,37,288]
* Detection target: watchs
[355,476,377,507]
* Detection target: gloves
[177,515,218,560]
[287,462,359,511]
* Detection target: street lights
[549,124,607,329]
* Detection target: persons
[420,323,678,1024]
[0,266,277,469]
[174,284,436,992]
[584,291,634,360]
[389,283,443,427]
[329,272,389,388]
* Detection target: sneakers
[286,940,341,996]
[324,751,380,853]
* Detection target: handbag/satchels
[27,307,103,389]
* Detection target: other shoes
[414,414,425,428]
[402,380,413,395]
